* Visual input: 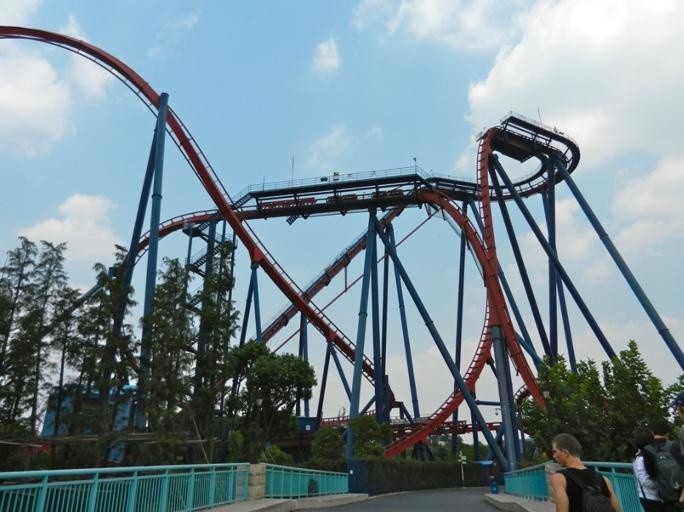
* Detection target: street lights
[456,450,467,489]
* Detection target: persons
[550,433,620,512]
[643,421,684,512]
[675,392,684,457]
[490,457,504,494]
[633,440,667,512]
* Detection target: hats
[672,392,684,406]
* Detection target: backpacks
[557,468,614,512]
[644,442,684,504]
